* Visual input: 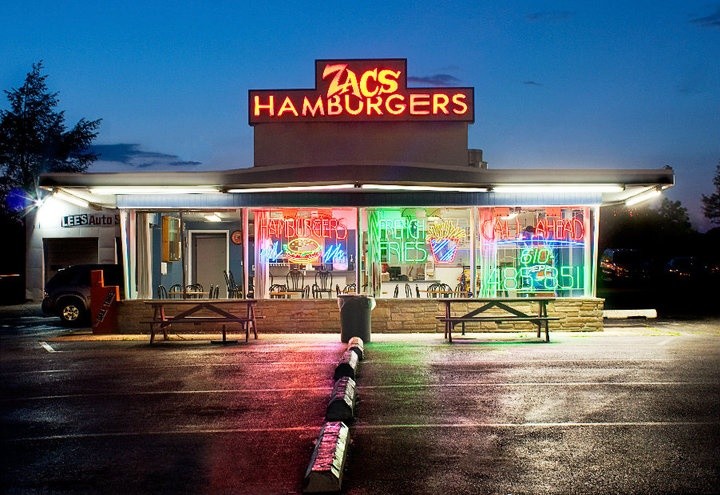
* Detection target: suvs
[40,264,123,327]
[598,248,647,287]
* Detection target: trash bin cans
[337,294,373,343]
[0,276,28,306]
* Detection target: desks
[420,291,468,298]
[435,297,556,343]
[269,291,299,299]
[341,291,356,293]
[168,291,209,300]
[144,299,260,343]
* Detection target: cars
[665,256,720,285]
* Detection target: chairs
[157,270,473,298]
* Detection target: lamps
[52,188,91,209]
[625,186,663,207]
[221,184,625,193]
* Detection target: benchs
[435,316,560,322]
[139,315,266,328]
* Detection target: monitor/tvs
[389,267,401,281]
